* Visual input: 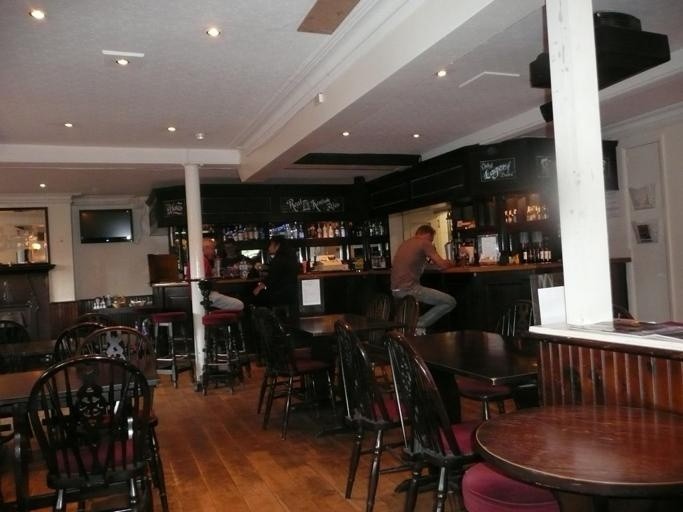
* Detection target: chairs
[333,317,427,511]
[254,304,333,442]
[452,301,536,416]
[73,326,160,448]
[49,323,107,365]
[391,293,421,335]
[26,354,169,512]
[380,330,483,512]
[362,295,393,320]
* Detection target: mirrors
[1,205,56,268]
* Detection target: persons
[222,242,253,273]
[186,238,243,315]
[244,234,298,352]
[390,222,458,339]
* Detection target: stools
[198,313,243,397]
[211,307,255,384]
[459,460,562,512]
[150,311,196,389]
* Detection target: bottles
[504,205,551,262]
[218,219,385,273]
[83,292,155,310]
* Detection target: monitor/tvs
[79,208,134,243]
